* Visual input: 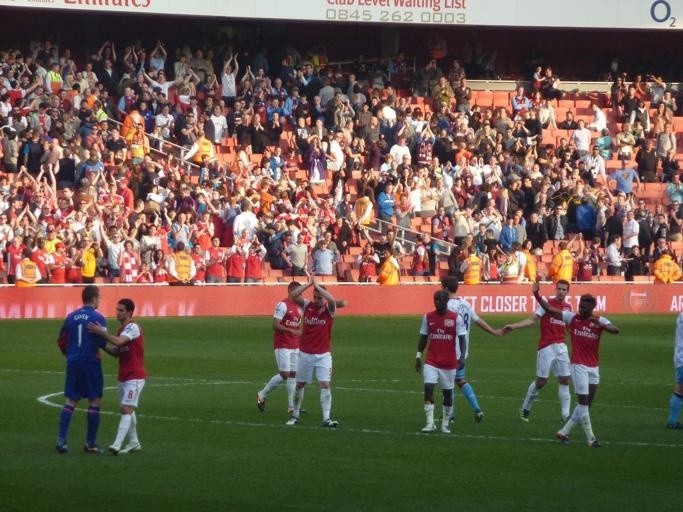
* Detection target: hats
[0,33,683,263]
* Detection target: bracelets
[286,327,294,334]
[414,350,422,361]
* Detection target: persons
[85,298,142,455]
[530,271,619,449]
[55,284,128,456]
[414,289,466,436]
[283,266,340,427]
[437,276,508,425]
[503,278,575,425]
[666,312,683,429]
[1,35,683,285]
[256,282,347,416]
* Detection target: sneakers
[120,441,142,454]
[474,411,484,423]
[84,442,104,454]
[421,423,437,433]
[55,437,70,454]
[585,437,604,448]
[560,415,573,425]
[322,418,340,428]
[555,430,569,443]
[666,421,683,430]
[519,406,530,422]
[257,392,266,411]
[440,426,453,435]
[108,440,119,454]
[439,413,456,423]
[288,407,307,414]
[285,415,300,427]
